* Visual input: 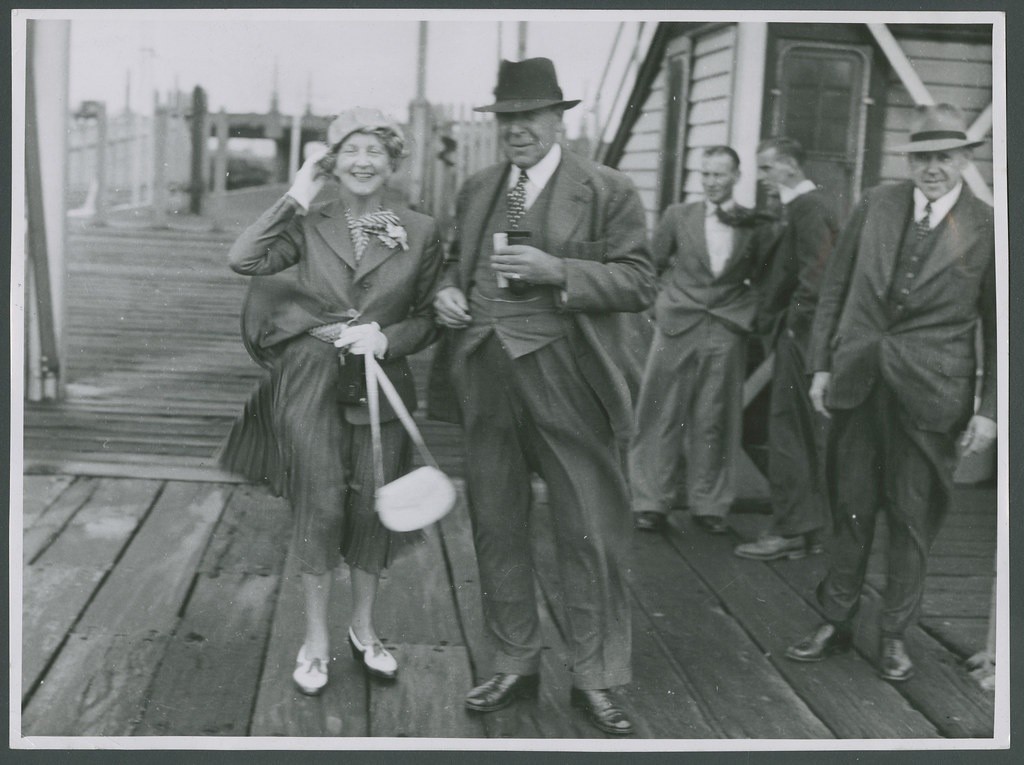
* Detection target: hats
[889,102,986,151]
[473,57,582,112]
[327,104,405,150]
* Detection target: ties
[716,208,779,225]
[916,202,932,241]
[506,170,527,229]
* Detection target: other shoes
[350,625,399,678]
[293,644,331,695]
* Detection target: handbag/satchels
[377,466,457,532]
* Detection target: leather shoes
[808,532,827,554]
[876,637,915,680]
[694,515,730,533]
[785,623,852,661]
[570,685,632,733]
[465,673,523,712]
[633,512,667,529]
[733,534,806,560]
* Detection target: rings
[514,274,521,281]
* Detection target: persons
[787,104,997,681]
[630,146,754,532]
[425,57,654,731]
[227,109,445,696]
[733,137,840,560]
